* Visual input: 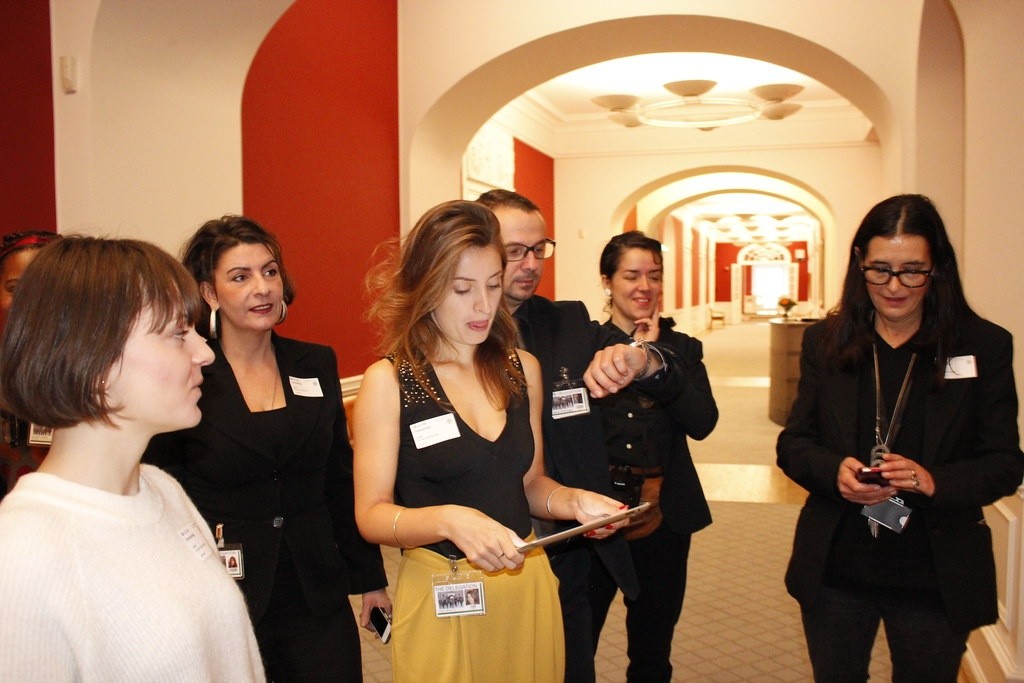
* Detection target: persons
[476,188,720,683]
[139,214,394,682]
[354,200,630,683]
[229,557,237,568]
[775,193,1024,683]
[0,220,268,683]
[0,229,55,498]
[574,395,583,403]
[466,590,480,605]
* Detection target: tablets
[516,501,651,555]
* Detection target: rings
[906,470,917,479]
[913,479,920,488]
[389,614,391,619]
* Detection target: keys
[868,520,878,536]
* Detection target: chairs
[708,309,726,329]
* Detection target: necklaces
[271,367,278,410]
[870,343,916,466]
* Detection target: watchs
[630,337,651,378]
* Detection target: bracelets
[546,486,567,518]
[394,507,414,548]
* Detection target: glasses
[858,255,936,287]
[505,238,556,262]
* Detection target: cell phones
[856,468,891,488]
[369,606,393,644]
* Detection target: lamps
[597,79,804,131]
